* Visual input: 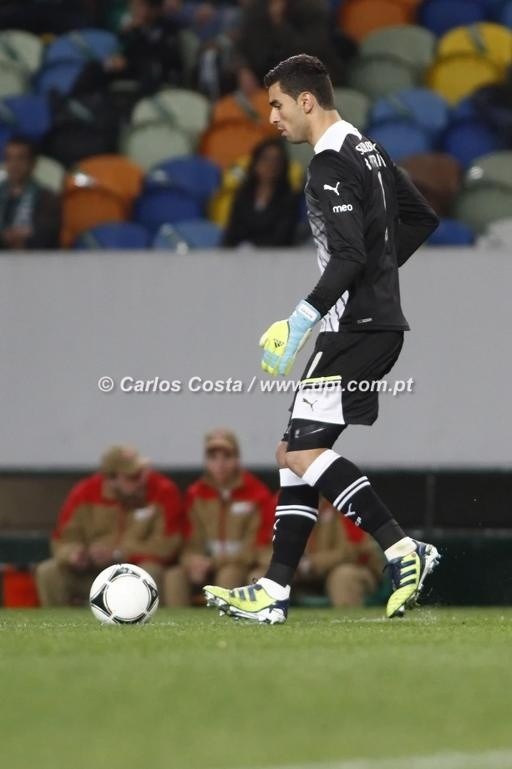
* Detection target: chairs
[0,0,510,247]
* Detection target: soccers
[90,565,158,626]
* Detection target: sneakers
[200,577,294,621]
[382,536,443,619]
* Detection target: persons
[202,54,443,627]
[249,489,387,609]
[1,1,512,252]
[36,446,184,610]
[163,430,273,610]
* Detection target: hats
[100,443,147,478]
[202,428,238,455]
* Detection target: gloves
[256,297,323,379]
